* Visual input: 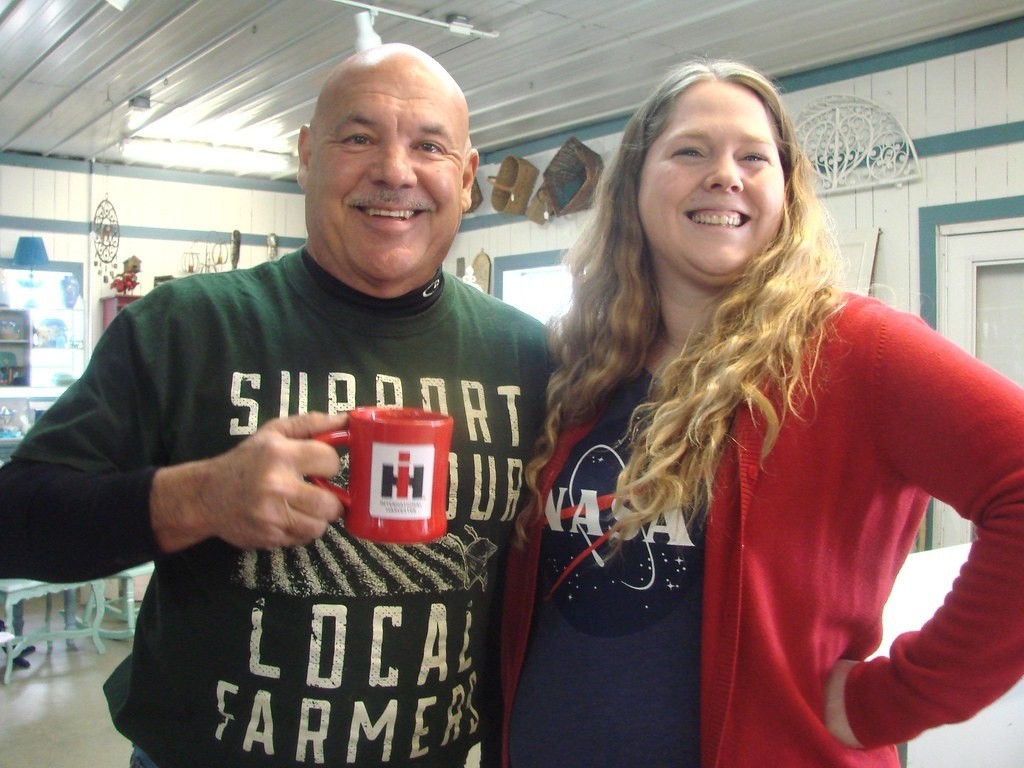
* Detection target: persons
[0,41,569,768]
[488,55,1023,768]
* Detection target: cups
[305,405,454,546]
